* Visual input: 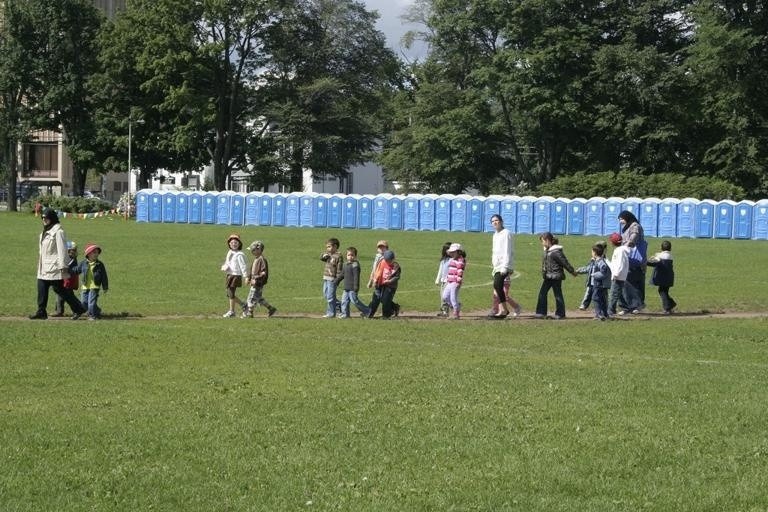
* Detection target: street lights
[125,118,146,217]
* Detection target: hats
[376,239,389,249]
[226,234,243,248]
[607,232,623,244]
[246,240,265,253]
[84,243,102,258]
[446,243,463,253]
[65,240,77,252]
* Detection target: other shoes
[665,310,671,314]
[322,312,336,317]
[454,311,459,317]
[395,304,400,316]
[489,309,497,316]
[338,313,350,318]
[579,304,585,311]
[553,314,561,319]
[496,310,509,317]
[438,312,448,317]
[361,312,368,317]
[619,310,628,316]
[534,313,544,317]
[513,306,521,317]
[632,309,640,315]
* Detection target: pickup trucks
[0,178,39,207]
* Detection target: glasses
[41,216,51,219]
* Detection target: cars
[61,189,104,201]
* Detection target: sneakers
[266,306,277,317]
[240,306,254,319]
[222,310,236,318]
[27,303,102,322]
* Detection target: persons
[488,270,522,320]
[441,242,465,320]
[333,246,369,318]
[360,240,388,319]
[531,231,577,319]
[238,241,277,318]
[490,213,514,319]
[433,243,452,317]
[374,250,400,320]
[27,206,86,321]
[574,209,678,319]
[317,238,343,319]
[47,239,79,317]
[220,234,250,318]
[68,242,108,320]
